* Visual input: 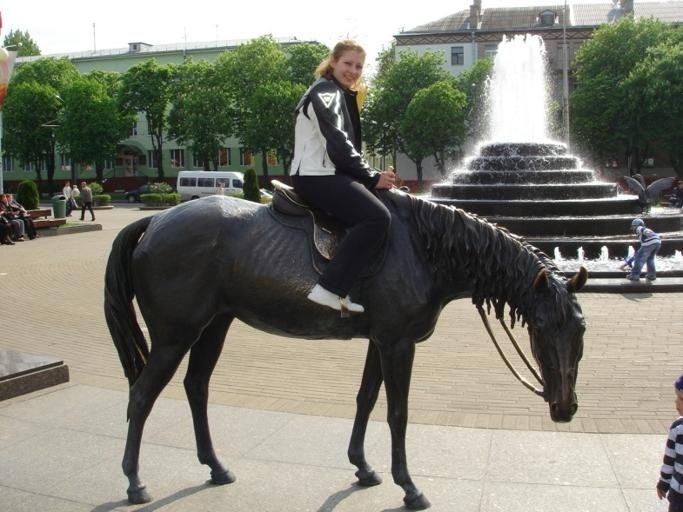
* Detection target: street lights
[55,94,75,185]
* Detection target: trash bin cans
[642,159,655,168]
[52,195,67,218]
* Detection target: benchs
[23,209,66,230]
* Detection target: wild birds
[622,174,674,202]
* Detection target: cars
[123,183,173,204]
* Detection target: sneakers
[626,273,640,281]
[0,235,40,245]
[645,274,656,280]
[307,282,365,313]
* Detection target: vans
[177,171,274,204]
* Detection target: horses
[103,186,589,510]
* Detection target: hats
[631,218,645,226]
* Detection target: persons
[669,180,683,208]
[0,193,40,245]
[72,185,80,196]
[625,218,662,281]
[288,40,398,314]
[78,182,95,221]
[62,181,73,217]
[656,374,683,511]
